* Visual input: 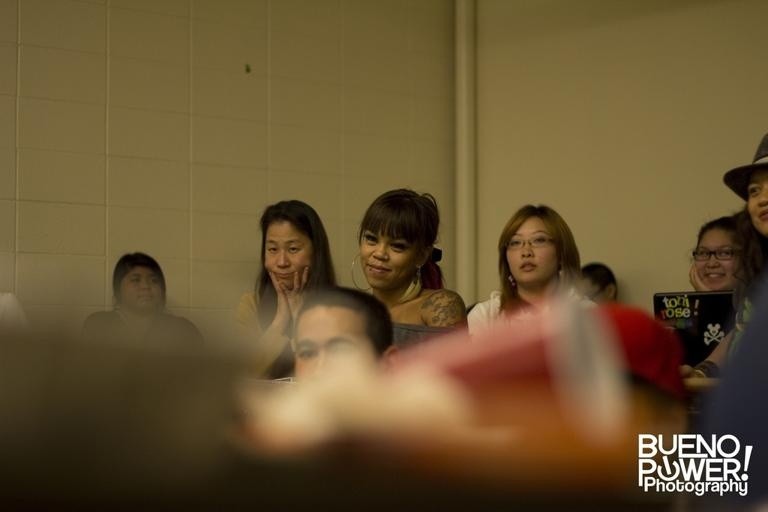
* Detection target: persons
[227,132,767,512]
[69,252,207,360]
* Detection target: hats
[723,133,768,201]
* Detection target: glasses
[692,247,744,261]
[503,236,556,249]
